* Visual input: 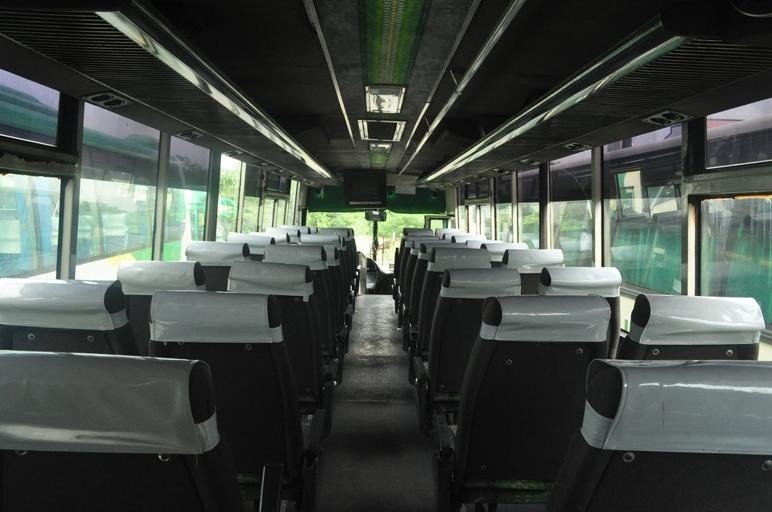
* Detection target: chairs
[547,359,770,511]
[0,224,359,410]
[147,289,330,512]
[431,294,614,511]
[1,348,284,511]
[392,226,771,438]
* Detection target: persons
[372,94,393,113]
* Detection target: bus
[473,101,772,360]
[1,85,223,305]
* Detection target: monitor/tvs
[343,169,387,209]
